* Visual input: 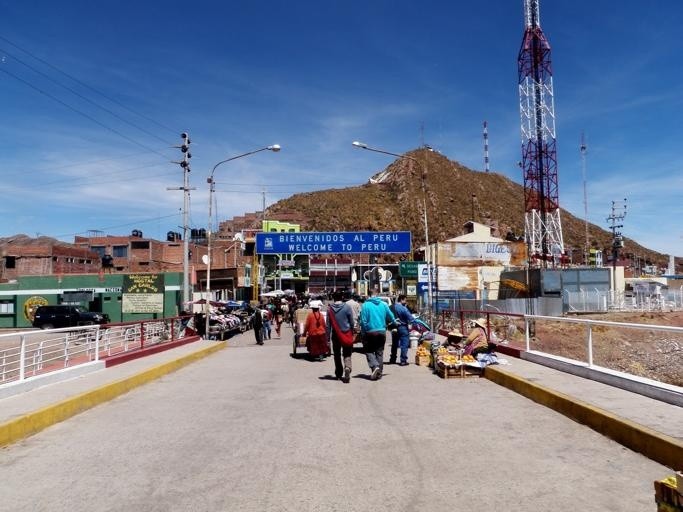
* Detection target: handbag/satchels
[338,330,353,347]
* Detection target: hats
[473,318,486,329]
[448,329,463,338]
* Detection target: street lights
[351,141,434,333]
[204,144,282,341]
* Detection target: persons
[326,292,355,385]
[303,302,329,365]
[387,295,422,365]
[463,318,490,357]
[360,288,402,380]
[248,289,354,346]
[345,295,361,342]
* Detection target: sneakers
[401,361,409,366]
[344,367,351,383]
[371,366,382,380]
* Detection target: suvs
[32,305,111,332]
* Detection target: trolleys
[292,326,332,357]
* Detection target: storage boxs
[415,340,433,366]
